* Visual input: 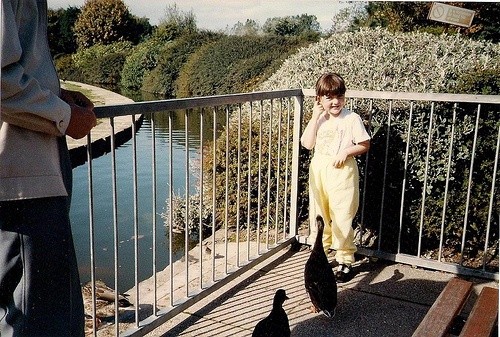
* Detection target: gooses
[303,215,340,320]
[251,289,291,337]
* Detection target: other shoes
[335,263,352,282]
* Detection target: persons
[301,72,371,283]
[0,0,97,337]
